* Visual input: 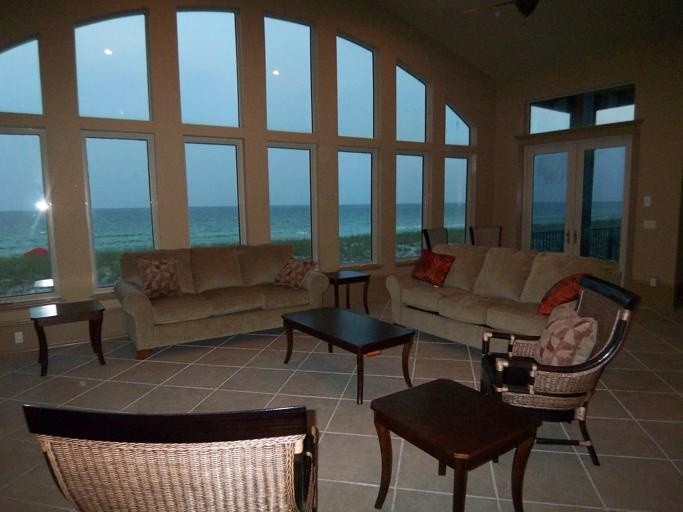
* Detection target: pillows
[271,253,320,292]
[135,252,183,301]
[535,271,593,318]
[410,248,455,287]
[532,297,598,367]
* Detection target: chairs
[467,225,503,248]
[479,273,642,511]
[421,226,449,249]
[21,401,320,512]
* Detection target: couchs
[111,242,331,361]
[383,242,623,355]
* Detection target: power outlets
[13,331,24,344]
[649,276,658,288]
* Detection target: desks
[321,268,371,316]
[280,307,416,405]
[368,377,546,512]
[29,298,107,376]
